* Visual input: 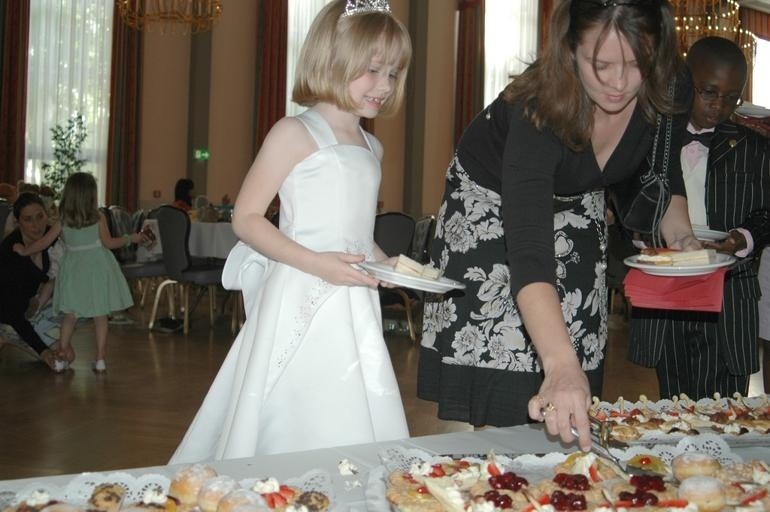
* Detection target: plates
[622,251,736,279]
[692,228,731,243]
[357,258,468,295]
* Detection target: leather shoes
[54,360,69,372]
[96,359,107,372]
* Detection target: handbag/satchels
[608,75,676,235]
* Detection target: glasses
[693,85,743,107]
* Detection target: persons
[624,35,770,402]
[13,173,143,373]
[417,0,702,451]
[167,0,413,462]
[39,186,58,215]
[0,193,64,373]
[171,178,195,210]
[5,183,40,238]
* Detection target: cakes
[370,253,441,281]
[635,247,717,268]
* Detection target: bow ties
[682,130,713,147]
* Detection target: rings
[540,402,555,417]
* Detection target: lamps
[671,0,758,64]
[116,0,224,41]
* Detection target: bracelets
[124,233,132,247]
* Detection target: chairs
[97,204,280,340]
[375,210,435,346]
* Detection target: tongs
[562,414,678,486]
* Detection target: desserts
[588,399,770,441]
[386,452,770,512]
[3,462,331,512]
